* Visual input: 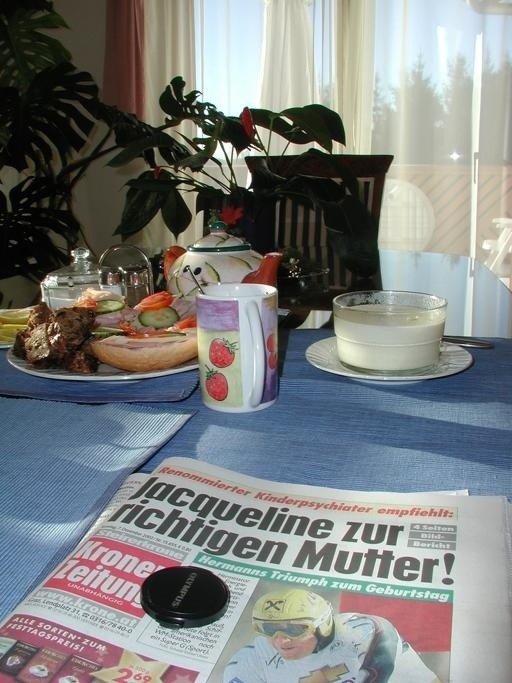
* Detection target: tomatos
[139,291,174,310]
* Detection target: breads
[91,327,198,372]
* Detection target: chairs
[245,155,394,317]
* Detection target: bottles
[40,249,102,314]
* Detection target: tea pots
[164,221,283,302]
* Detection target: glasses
[251,601,332,640]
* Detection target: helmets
[250,588,335,653]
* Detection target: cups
[197,283,280,415]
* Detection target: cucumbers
[93,299,124,315]
[90,325,126,338]
[139,305,180,329]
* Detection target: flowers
[103,102,381,278]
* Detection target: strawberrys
[209,337,239,369]
[269,354,278,369]
[267,334,277,353]
[205,364,229,402]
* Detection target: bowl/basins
[333,289,446,376]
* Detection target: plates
[305,337,476,388]
[7,346,197,383]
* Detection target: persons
[226,586,441,681]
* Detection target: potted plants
[0,0,113,308]
